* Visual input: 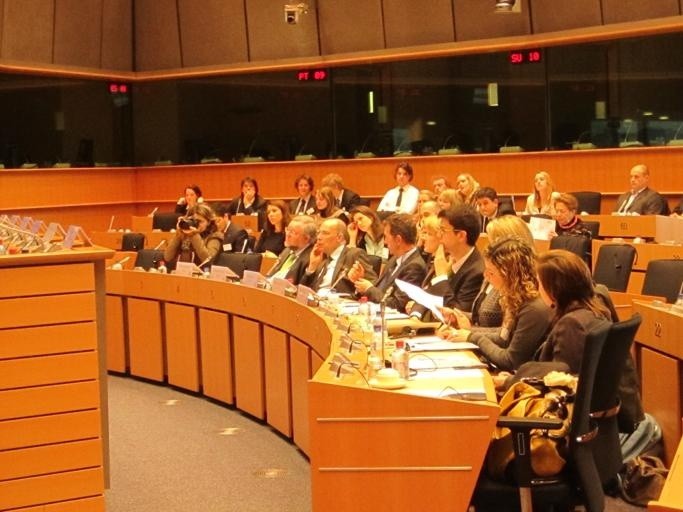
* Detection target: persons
[255,161,593,340]
[226,176,270,216]
[174,185,204,215]
[614,164,671,216]
[436,234,555,371]
[492,249,646,436]
[163,204,224,273]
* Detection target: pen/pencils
[446,318,451,330]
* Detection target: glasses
[439,227,461,234]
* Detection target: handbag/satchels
[616,455,669,506]
[487,382,566,479]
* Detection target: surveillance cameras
[284,3,309,27]
[492,0,523,14]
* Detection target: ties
[396,189,403,206]
[484,217,489,232]
[280,253,295,271]
[377,259,397,290]
[299,199,306,214]
[313,256,333,293]
[335,199,339,206]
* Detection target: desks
[0,226,116,512]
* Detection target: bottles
[391,341,408,376]
[157,260,167,274]
[357,297,370,317]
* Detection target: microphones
[153,239,167,250]
[149,206,159,216]
[241,239,249,254]
[0,223,51,252]
[108,256,131,269]
[106,216,115,233]
[380,285,395,368]
[319,267,350,306]
[193,255,213,275]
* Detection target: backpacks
[618,412,661,465]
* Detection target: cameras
[179,215,200,231]
